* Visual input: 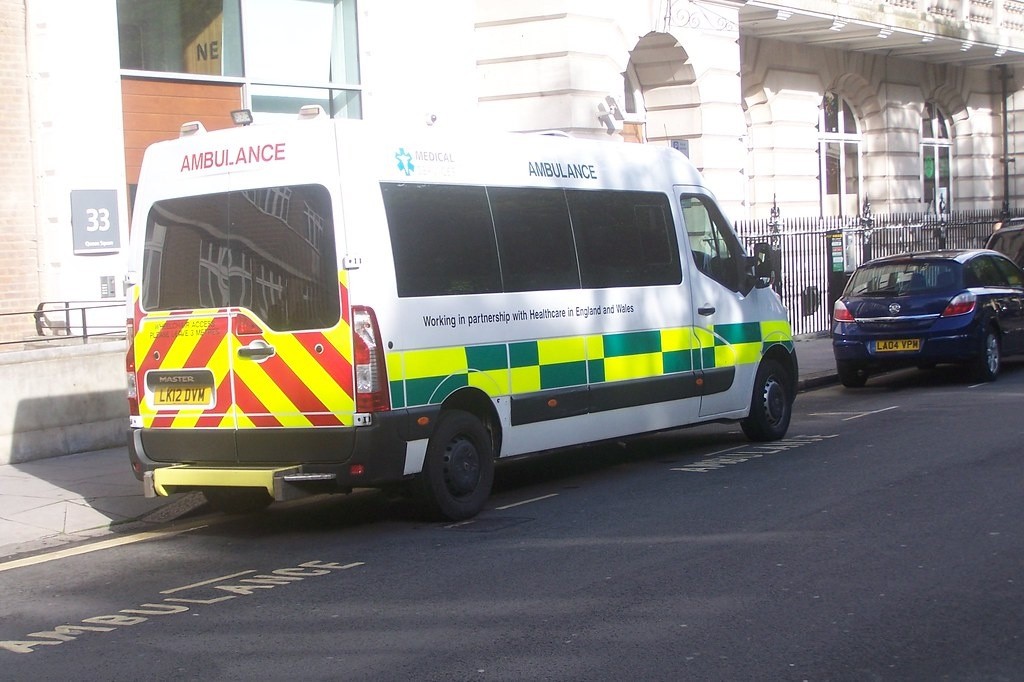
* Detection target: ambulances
[123,105,800,525]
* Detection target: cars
[832,247,1024,388]
[984,218,1023,270]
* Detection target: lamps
[596,95,627,121]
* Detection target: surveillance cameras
[426,114,437,125]
[605,95,627,120]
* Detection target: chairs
[937,271,955,287]
[911,273,926,289]
